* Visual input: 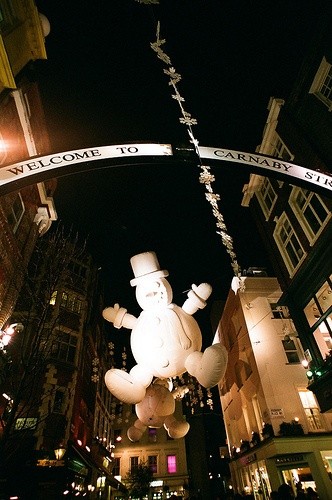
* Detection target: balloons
[98,250,231,443]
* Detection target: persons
[15,477,332,500]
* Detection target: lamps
[302,360,316,380]
